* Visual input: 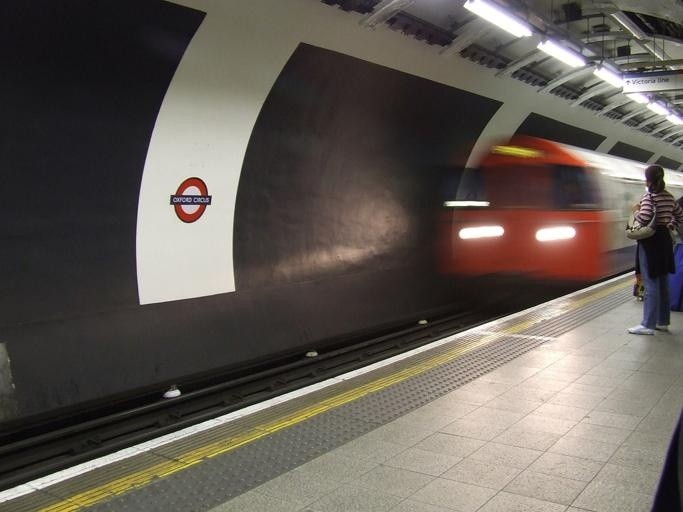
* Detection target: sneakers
[627,323,669,335]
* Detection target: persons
[627,166,683,336]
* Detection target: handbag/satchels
[625,206,657,240]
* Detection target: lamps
[462,0,683,128]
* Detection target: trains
[441,134,683,286]
